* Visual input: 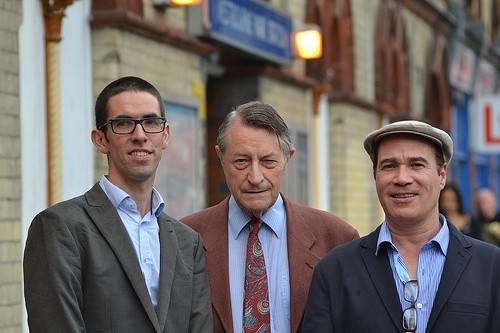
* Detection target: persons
[438,184,500,249]
[300,121,500,333]
[23,76,213,333]
[178,100,361,333]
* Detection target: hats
[363,121,453,167]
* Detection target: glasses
[98,117,166,134]
[402,280,419,331]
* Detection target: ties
[242,220,271,333]
